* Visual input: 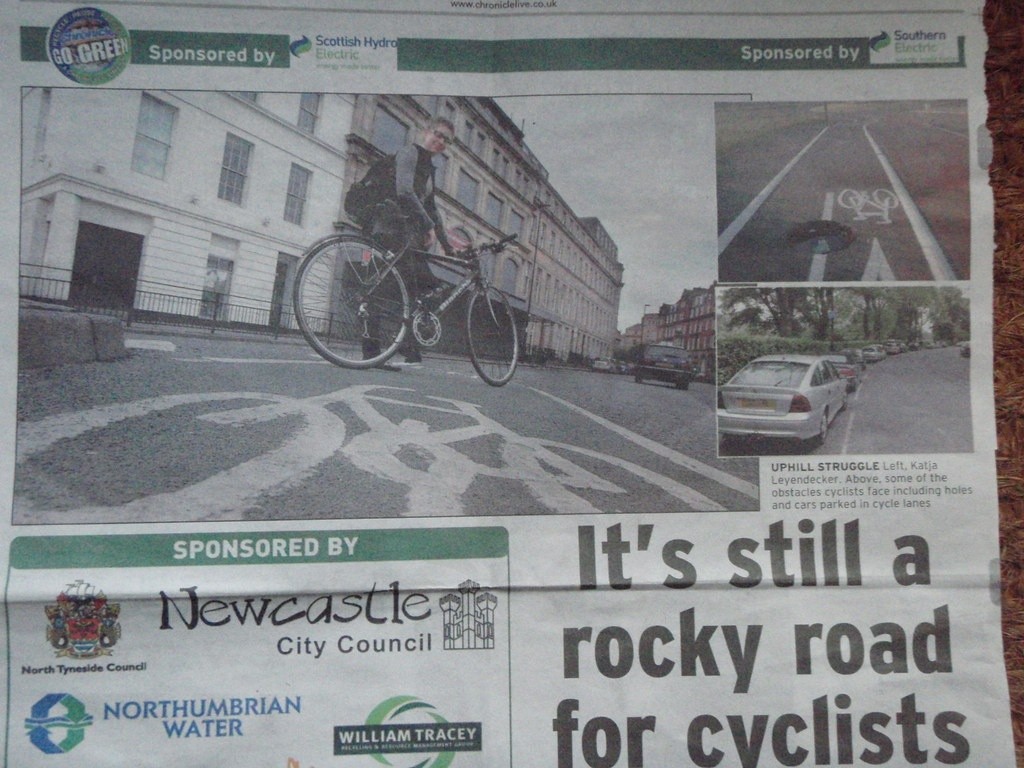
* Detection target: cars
[717,352,858,447]
[847,339,970,394]
[592,356,634,375]
[634,344,698,391]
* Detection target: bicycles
[290,199,521,388]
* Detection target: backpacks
[343,146,397,227]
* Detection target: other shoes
[361,348,401,371]
[386,338,423,364]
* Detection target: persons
[611,358,639,373]
[345,117,455,371]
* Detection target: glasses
[430,130,452,145]
